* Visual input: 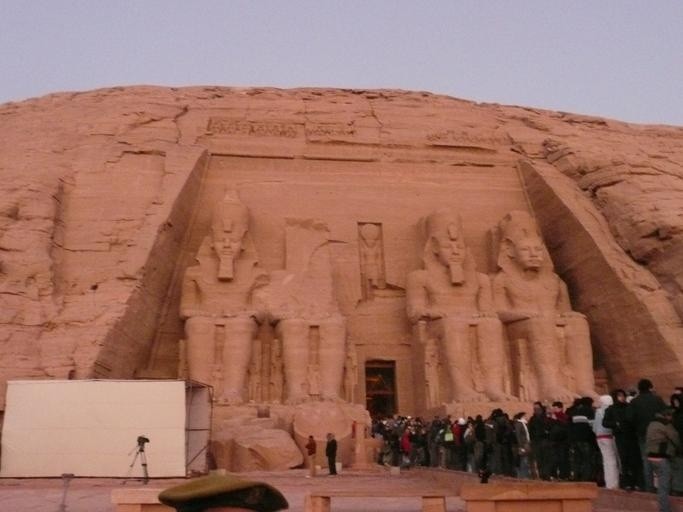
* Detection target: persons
[178,190,269,405]
[493,211,599,404]
[372,407,537,484]
[404,209,519,402]
[275,319,348,402]
[305,435,316,478]
[519,378,683,510]
[325,433,337,477]
[359,237,381,277]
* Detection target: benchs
[110,488,176,512]
[309,487,448,512]
[458,482,598,512]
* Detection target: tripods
[122,447,149,484]
